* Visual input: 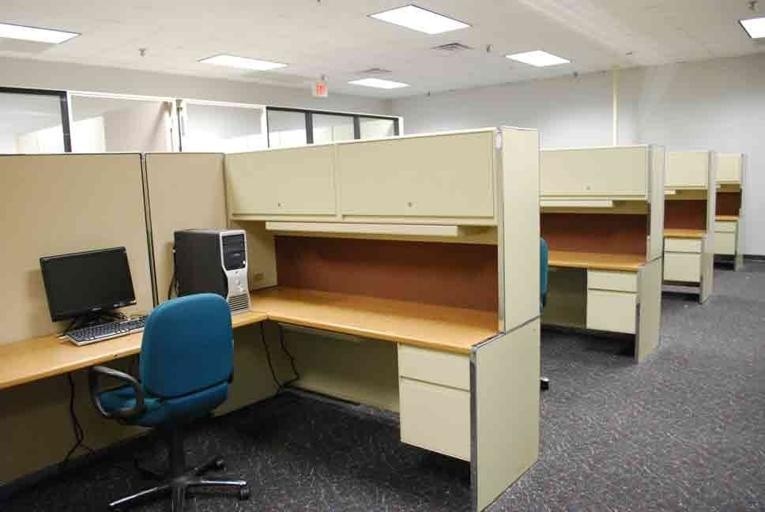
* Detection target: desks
[663,228,714,304]
[714,215,744,272]
[548,249,663,365]
[0,281,539,509]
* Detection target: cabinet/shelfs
[224,141,333,221]
[716,153,743,186]
[541,145,665,202]
[334,129,541,222]
[665,150,717,192]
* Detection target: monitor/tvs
[39,246,137,336]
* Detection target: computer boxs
[174,228,251,317]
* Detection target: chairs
[539,237,551,391]
[87,294,252,512]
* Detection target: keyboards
[65,315,149,347]
